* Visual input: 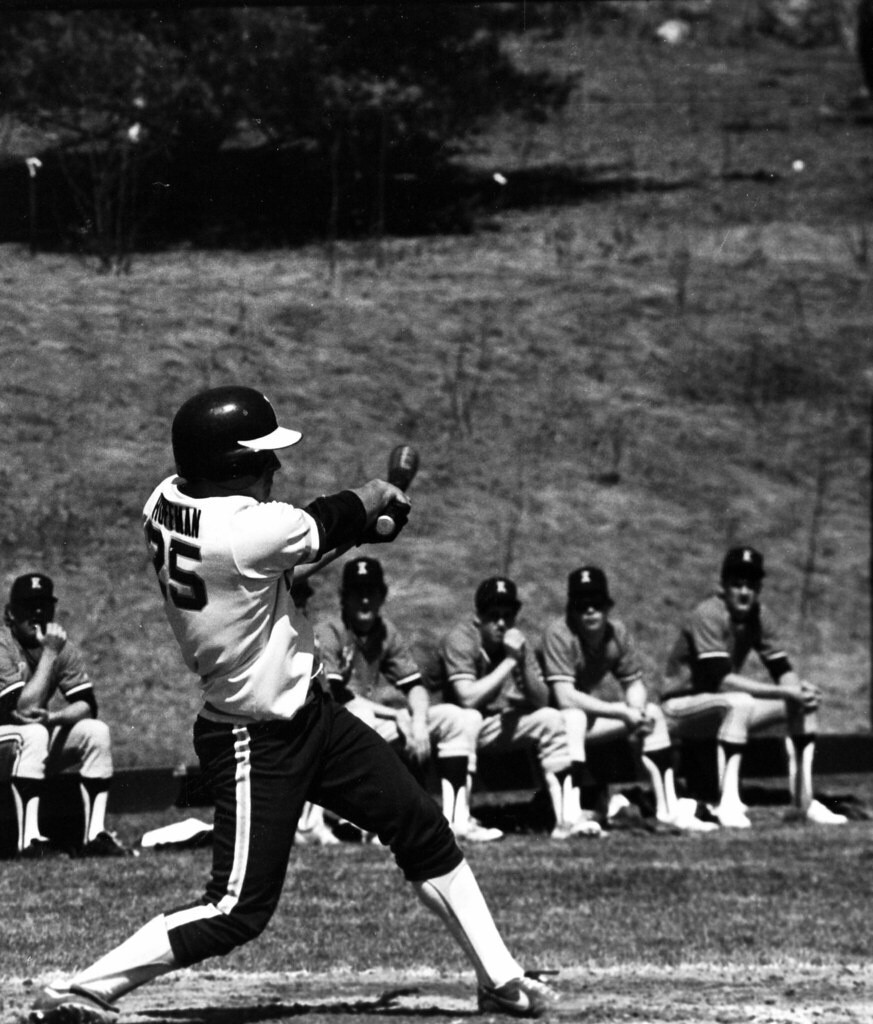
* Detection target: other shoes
[791,798,852,826]
[545,820,609,843]
[708,805,750,830]
[653,811,721,835]
[82,832,126,856]
[451,816,503,843]
[12,836,65,861]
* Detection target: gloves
[357,499,412,547]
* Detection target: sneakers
[30,983,121,1024]
[477,969,579,1019]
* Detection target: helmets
[564,567,610,605]
[8,572,59,607]
[171,386,304,479]
[343,556,385,594]
[721,544,767,580]
[476,577,523,618]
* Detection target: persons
[658,545,848,829]
[23,386,564,1024]
[0,574,140,859]
[417,574,601,843]
[540,564,718,837]
[291,555,503,843]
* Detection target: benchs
[0,725,873,859]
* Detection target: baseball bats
[374,442,419,536]
